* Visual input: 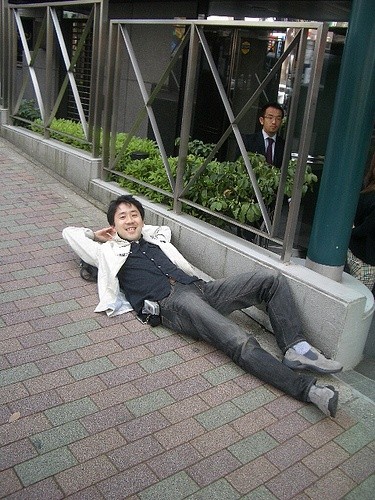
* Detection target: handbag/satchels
[79,260,98,283]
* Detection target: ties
[266,138,275,164]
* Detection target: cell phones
[144,300,159,315]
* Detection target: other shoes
[307,384,338,418]
[282,345,343,373]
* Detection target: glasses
[262,115,282,122]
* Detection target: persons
[62,193,344,419]
[346,147,375,290]
[236,102,290,240]
